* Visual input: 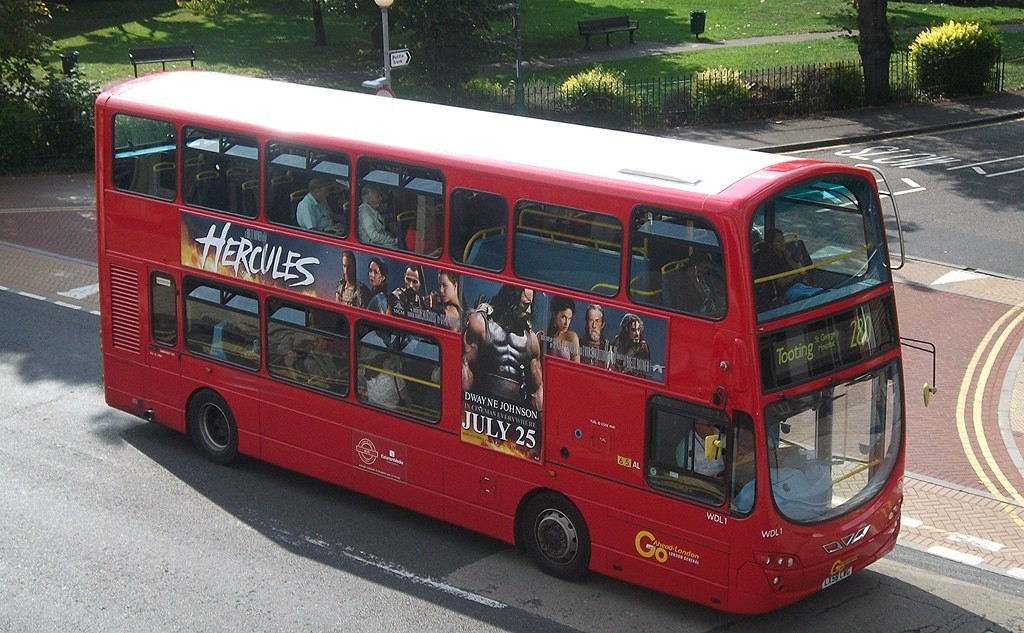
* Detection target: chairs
[120,151,831,499]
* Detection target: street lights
[373,0,396,100]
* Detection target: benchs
[578,15,639,50]
[128,47,196,78]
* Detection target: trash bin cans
[690,10,707,34]
[58,51,79,73]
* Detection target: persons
[357,184,399,247]
[543,294,581,362]
[610,313,652,381]
[762,227,824,303]
[460,282,543,449]
[676,417,728,482]
[333,250,372,309]
[578,303,614,371]
[671,246,727,318]
[385,262,428,323]
[192,287,443,412]
[295,175,343,234]
[428,269,464,332]
[367,256,392,314]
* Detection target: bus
[93,67,939,615]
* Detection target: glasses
[697,423,713,428]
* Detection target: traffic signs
[389,47,412,67]
